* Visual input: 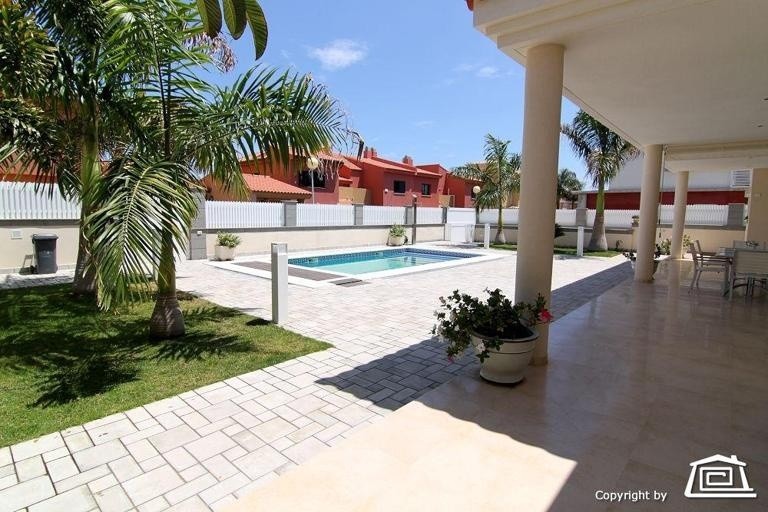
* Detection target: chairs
[686,238,768,302]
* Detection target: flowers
[621,241,663,262]
[426,285,555,363]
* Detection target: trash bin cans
[32,232,58,274]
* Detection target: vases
[467,322,540,388]
[630,260,659,280]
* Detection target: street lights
[305,156,319,203]
[472,185,481,200]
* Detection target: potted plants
[215,231,241,261]
[388,221,409,246]
[681,234,692,258]
